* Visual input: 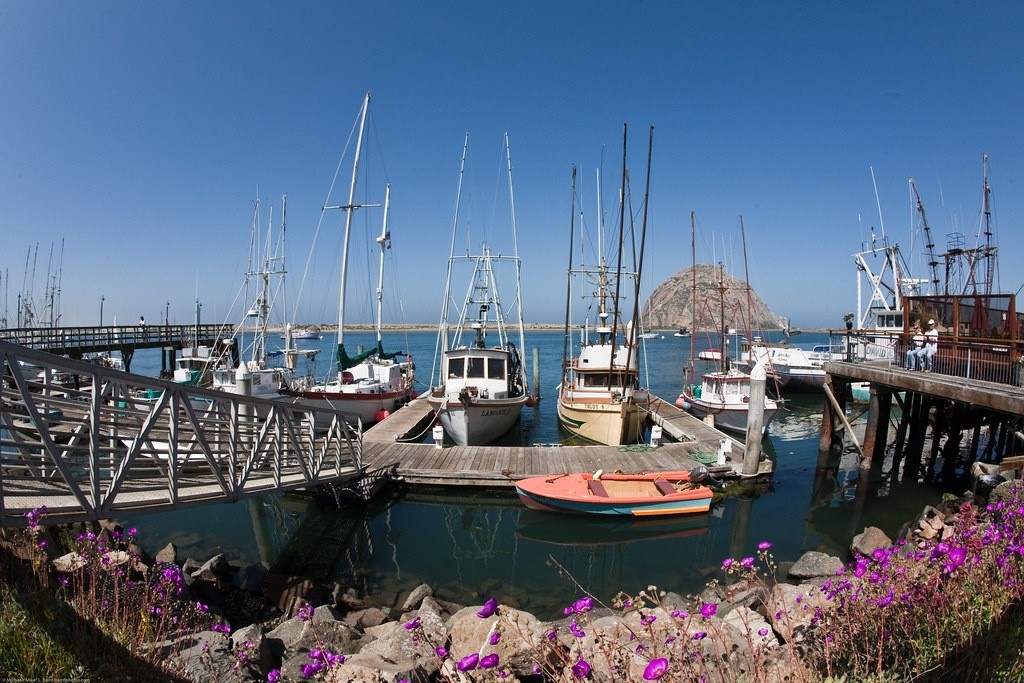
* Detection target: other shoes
[904,367,915,371]
[921,369,932,373]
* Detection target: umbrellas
[971,297,989,338]
[1004,299,1019,333]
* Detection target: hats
[928,319,935,325]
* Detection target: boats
[513,465,732,518]
[516,496,729,546]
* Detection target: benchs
[898,338,1018,384]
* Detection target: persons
[904,326,924,371]
[917,319,938,373]
[139,316,146,341]
[960,326,965,336]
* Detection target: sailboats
[7,90,1023,448]
